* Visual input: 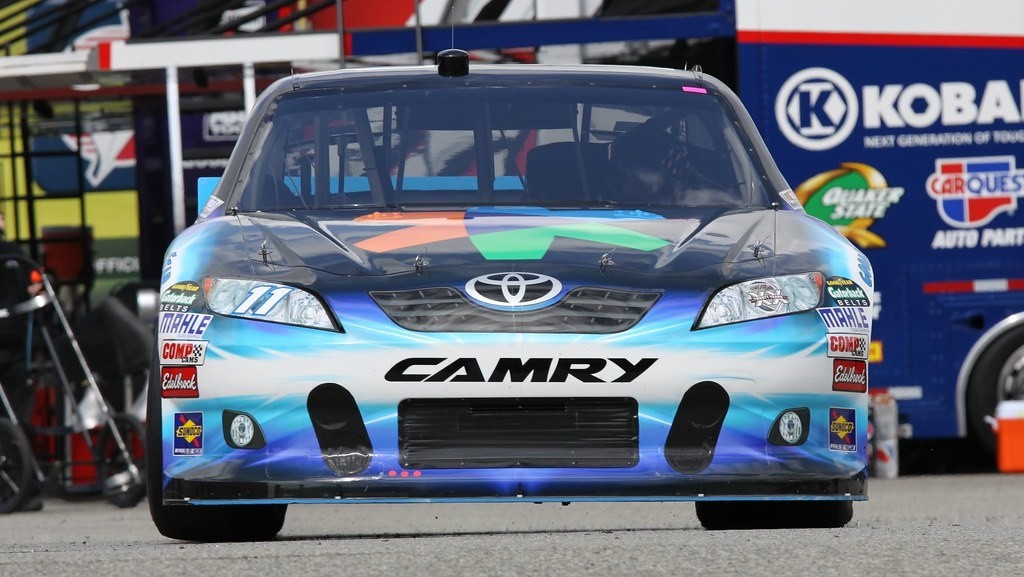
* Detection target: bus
[0,1,1024,456]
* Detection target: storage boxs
[984,400,1024,473]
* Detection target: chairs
[524,141,608,202]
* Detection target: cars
[143,48,876,542]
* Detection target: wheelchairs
[0,252,146,514]
